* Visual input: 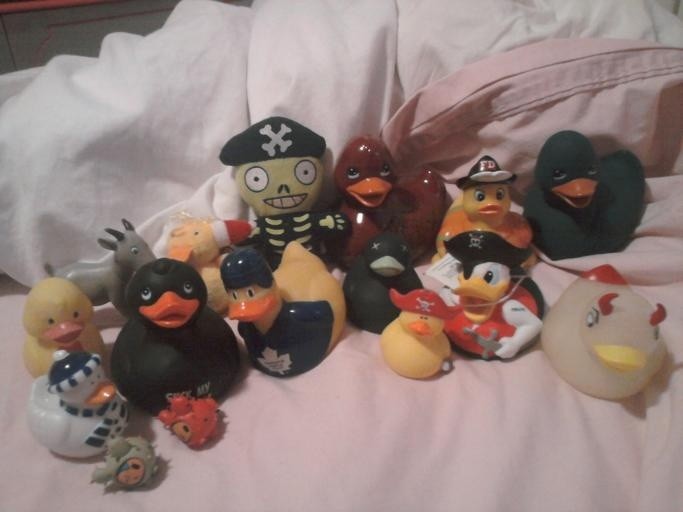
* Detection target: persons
[220,116,351,273]
